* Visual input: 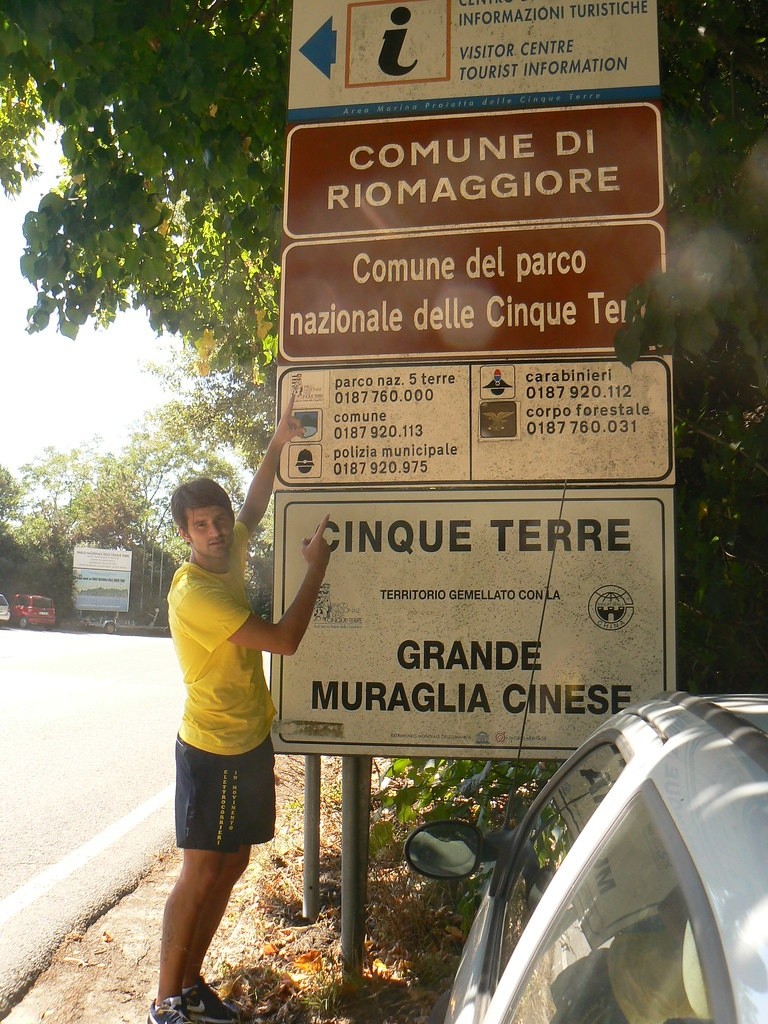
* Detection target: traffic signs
[289,2,664,122]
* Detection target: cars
[80,608,170,636]
[0,594,11,625]
[10,594,56,631]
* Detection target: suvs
[402,691,767,1023]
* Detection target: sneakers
[147,996,192,1024]
[181,977,242,1023]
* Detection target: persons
[146,394,330,1024]
